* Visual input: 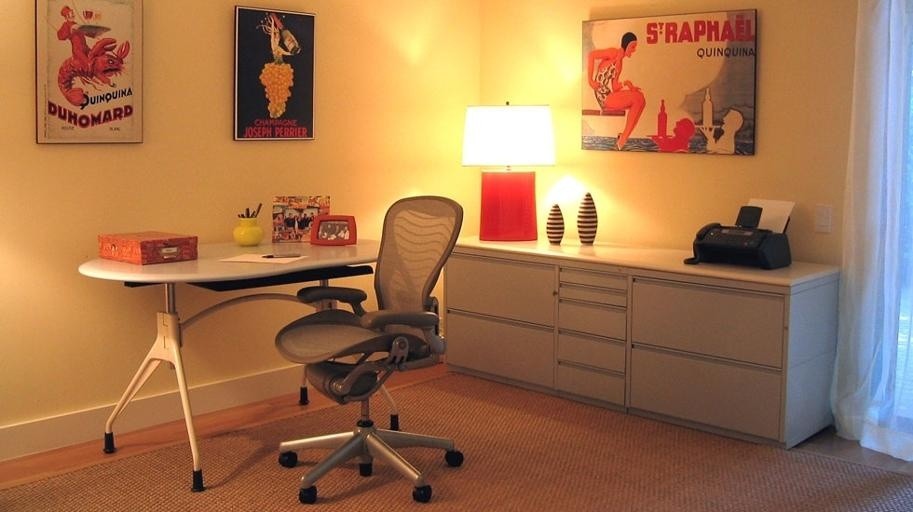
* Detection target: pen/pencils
[241,213,245,219]
[255,203,262,217]
[262,253,300,258]
[246,207,250,218]
[238,214,241,218]
[250,211,255,217]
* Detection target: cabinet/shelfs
[444,236,842,452]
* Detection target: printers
[683,198,793,271]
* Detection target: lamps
[461,105,556,242]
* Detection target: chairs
[275,196,464,503]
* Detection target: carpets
[0,371,912,512]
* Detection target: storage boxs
[98,231,198,265]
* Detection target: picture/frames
[272,193,330,242]
[235,6,317,140]
[311,215,356,245]
[35,0,143,144]
[581,8,756,155]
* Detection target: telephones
[684,206,791,270]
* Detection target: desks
[79,238,400,493]
